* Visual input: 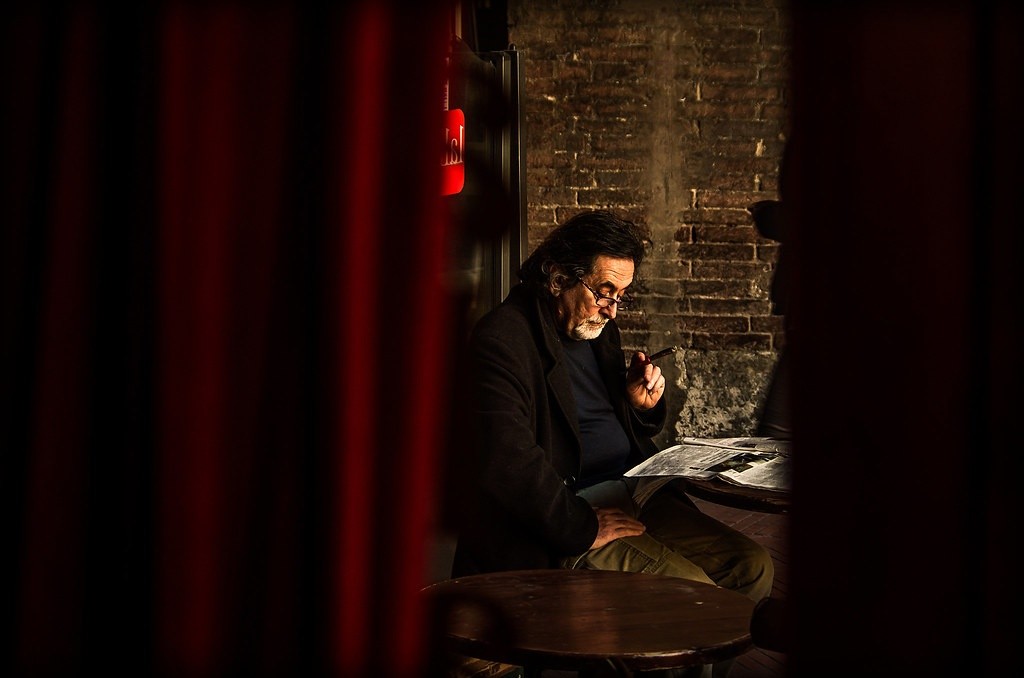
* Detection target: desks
[682,439,790,516]
[419,569,758,678]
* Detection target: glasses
[563,272,631,308]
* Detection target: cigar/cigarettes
[650,345,678,362]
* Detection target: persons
[445,208,775,677]
